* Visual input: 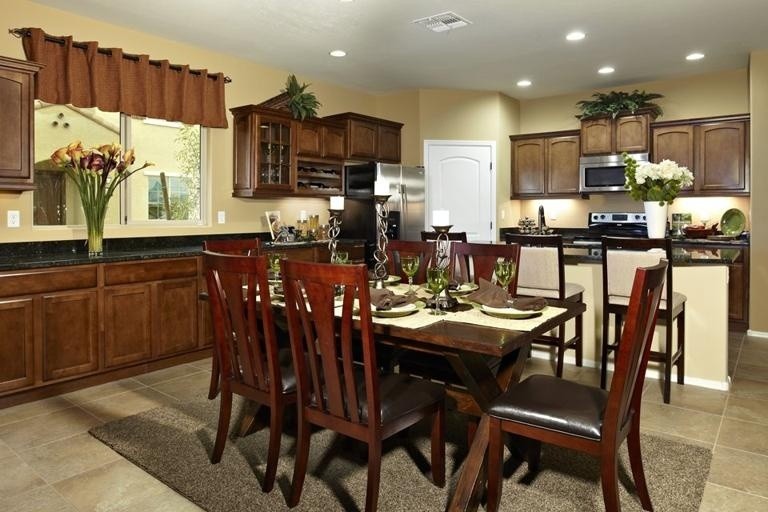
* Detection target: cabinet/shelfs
[0,55,47,190]
[230,104,343,198]
[509,129,589,200]
[575,110,658,156]
[725,247,749,332]
[322,112,404,163]
[101,253,198,383]
[650,113,750,197]
[0,262,101,409]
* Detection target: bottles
[297,214,329,240]
[298,166,341,188]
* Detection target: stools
[601,233,685,403]
[506,234,586,376]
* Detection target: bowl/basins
[434,225,453,234]
[686,228,716,238]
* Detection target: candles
[434,208,449,226]
[330,196,344,210]
[373,174,390,196]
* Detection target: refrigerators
[345,161,427,241]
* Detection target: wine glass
[494,260,515,294]
[331,251,351,302]
[400,255,447,315]
[269,252,283,287]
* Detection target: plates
[420,282,480,295]
[708,236,733,240]
[369,300,427,320]
[368,275,400,286]
[721,209,745,236]
[485,303,544,319]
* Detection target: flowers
[622,151,695,206]
[50,141,155,252]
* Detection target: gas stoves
[575,226,646,241]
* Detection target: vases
[80,207,108,256]
[644,201,668,239]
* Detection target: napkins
[368,285,418,311]
[470,279,547,312]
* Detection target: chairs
[203,250,344,494]
[401,243,522,400]
[204,238,286,402]
[328,240,437,372]
[484,258,670,512]
[278,256,448,510]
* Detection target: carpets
[88,390,713,512]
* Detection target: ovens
[579,153,650,193]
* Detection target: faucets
[537,204,547,233]
